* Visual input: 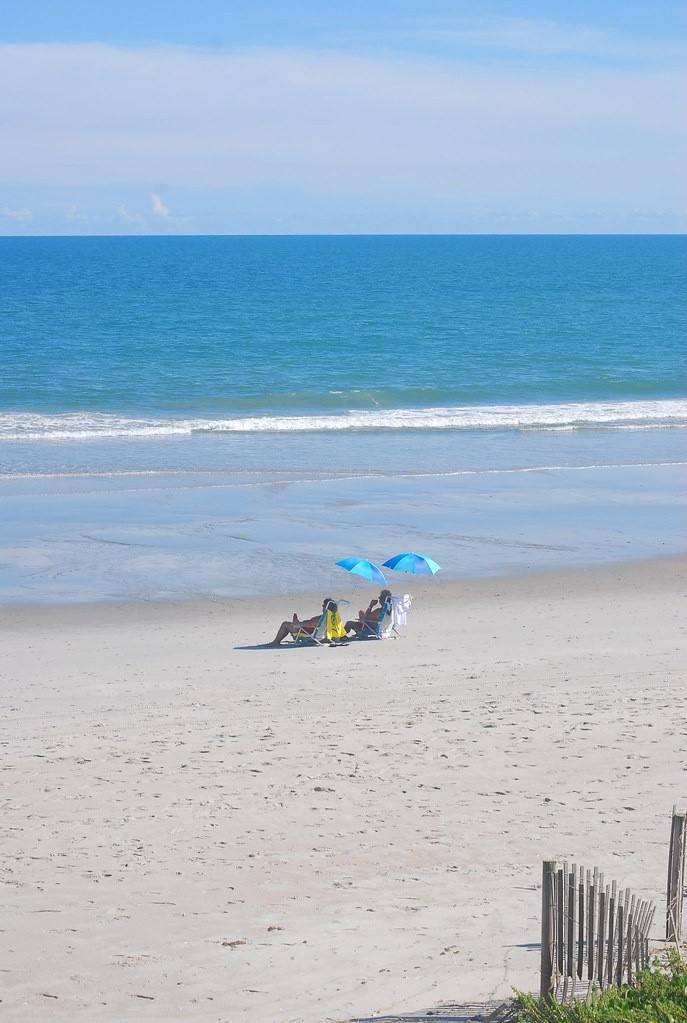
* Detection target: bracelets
[368,607,372,609]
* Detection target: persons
[344,590,392,633]
[265,599,335,646]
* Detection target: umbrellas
[335,557,389,593]
[381,552,442,575]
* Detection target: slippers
[336,642,349,646]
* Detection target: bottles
[294,612,297,619]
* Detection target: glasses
[378,597,384,600]
[322,605,326,607]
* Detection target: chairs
[290,599,350,647]
[349,599,407,640]
